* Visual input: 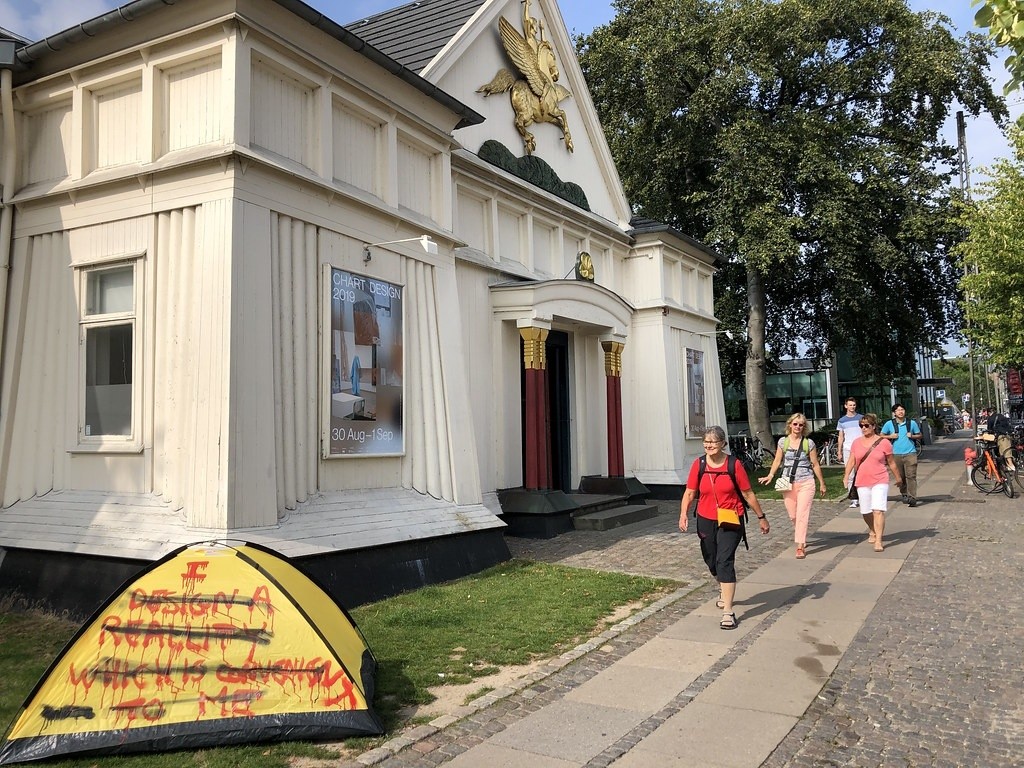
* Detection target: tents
[0,538,385,768]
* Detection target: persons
[967,419,973,429]
[879,403,923,506]
[954,415,964,430]
[758,413,826,558]
[987,407,1015,471]
[837,397,864,508]
[679,425,770,629]
[976,408,988,425]
[843,413,902,552]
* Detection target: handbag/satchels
[773,476,792,492]
[847,478,859,500]
[717,507,741,530]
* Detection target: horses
[511,42,574,156]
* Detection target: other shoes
[795,547,805,558]
[1004,465,1016,471]
[873,541,884,552]
[902,495,916,507]
[848,500,860,508]
[867,532,876,543]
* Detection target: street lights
[805,371,814,432]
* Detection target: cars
[955,415,964,429]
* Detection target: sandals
[716,591,725,608]
[719,612,738,630]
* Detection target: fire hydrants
[965,448,977,485]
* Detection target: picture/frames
[321,263,406,459]
[683,347,707,440]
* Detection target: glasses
[703,440,722,445]
[894,409,905,412]
[793,423,803,426]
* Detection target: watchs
[758,513,765,519]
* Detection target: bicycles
[1003,430,1024,491]
[915,440,922,457]
[730,429,775,472]
[819,433,845,466]
[971,439,1014,498]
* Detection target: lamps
[691,330,734,339]
[363,234,438,255]
